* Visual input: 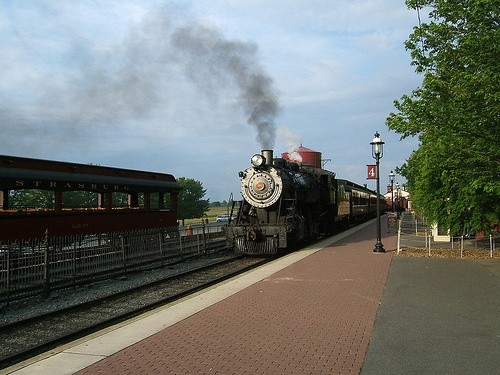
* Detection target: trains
[221,143,395,257]
[0,155,185,260]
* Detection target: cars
[216,215,234,222]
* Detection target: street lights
[369,130,386,252]
[388,169,396,213]
[396,181,401,220]
